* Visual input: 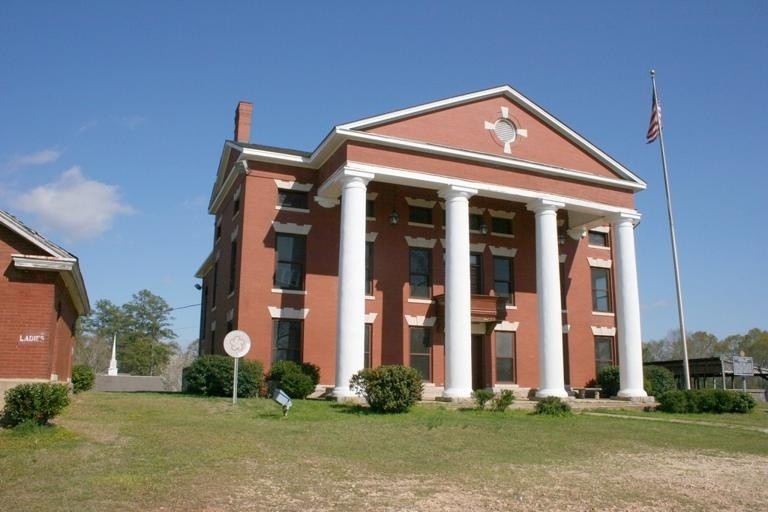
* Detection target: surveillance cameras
[578,230,587,240]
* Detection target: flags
[646,85,662,143]
